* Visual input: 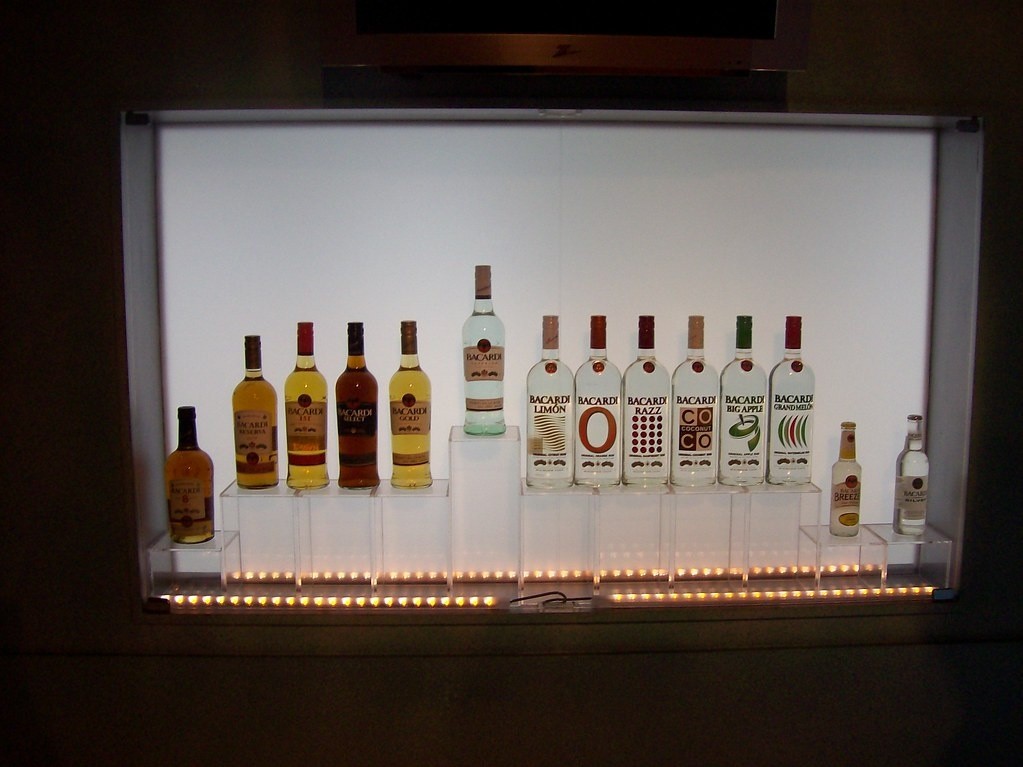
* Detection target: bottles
[622,315,672,487]
[672,315,720,488]
[526,315,576,490]
[830,422,863,537]
[461,265,506,436]
[720,316,769,487]
[388,320,433,490]
[892,415,929,535]
[232,335,280,489]
[284,322,331,491]
[164,407,215,544]
[575,314,621,488]
[335,322,380,489]
[769,315,816,486]
